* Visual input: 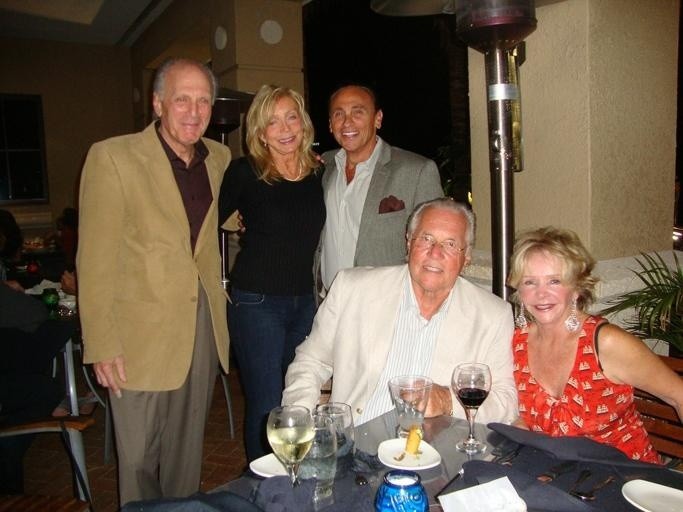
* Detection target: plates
[250,453,290,477]
[622,478,683,511]
[377,439,440,472]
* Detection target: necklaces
[284,161,302,182]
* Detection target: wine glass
[450,363,492,453]
[267,405,316,488]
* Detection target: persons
[75,57,241,508]
[505,226,683,466]
[279,198,519,428]
[237,84,446,307]
[1,208,98,495]
[217,85,327,470]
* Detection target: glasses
[411,234,470,255]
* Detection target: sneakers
[53,397,72,417]
[80,393,97,416]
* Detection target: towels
[251,475,376,512]
[487,422,667,471]
[120,491,264,512]
[460,461,599,512]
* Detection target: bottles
[41,288,59,309]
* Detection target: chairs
[633,354,683,476]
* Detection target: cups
[388,376,433,439]
[316,402,355,475]
[298,416,336,504]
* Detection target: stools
[0,414,96,503]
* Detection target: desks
[15,278,78,417]
